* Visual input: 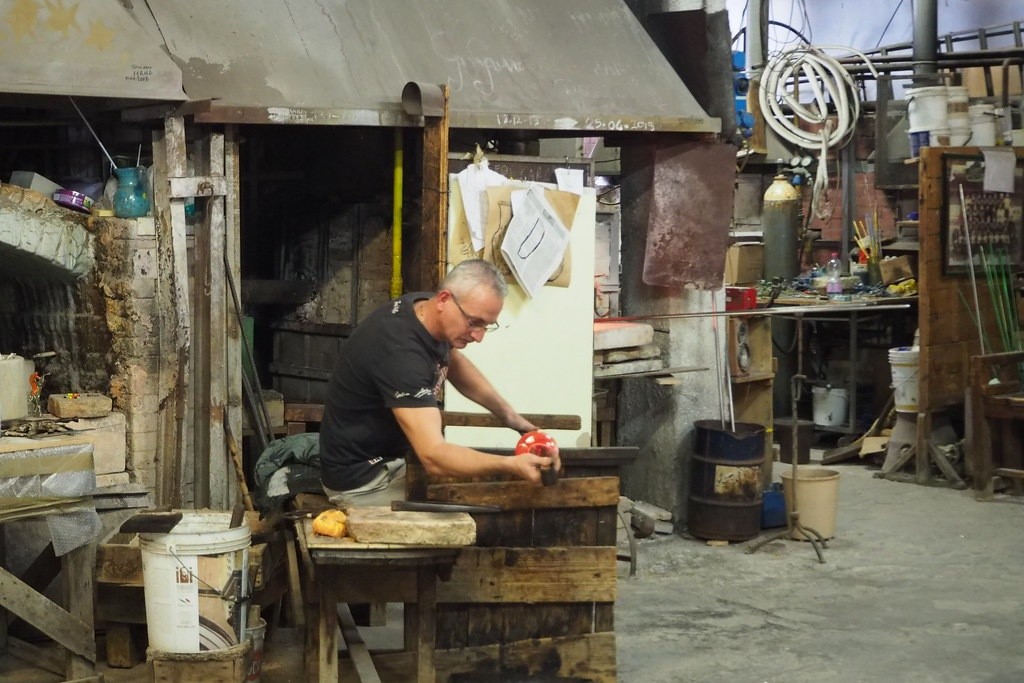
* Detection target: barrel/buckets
[247,618,267,683]
[888,346,919,412]
[907,86,1012,157]
[812,387,847,427]
[781,469,839,540]
[138,512,251,652]
[692,421,766,540]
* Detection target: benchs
[286,493,462,683]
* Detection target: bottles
[826,253,841,300]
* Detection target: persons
[320,261,561,506]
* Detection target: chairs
[969,352,1024,492]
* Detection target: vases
[111,166,151,217]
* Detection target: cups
[868,256,882,285]
[115,166,150,217]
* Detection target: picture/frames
[942,152,1024,277]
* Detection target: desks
[770,294,918,435]
[1,438,104,683]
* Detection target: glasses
[450,292,499,332]
